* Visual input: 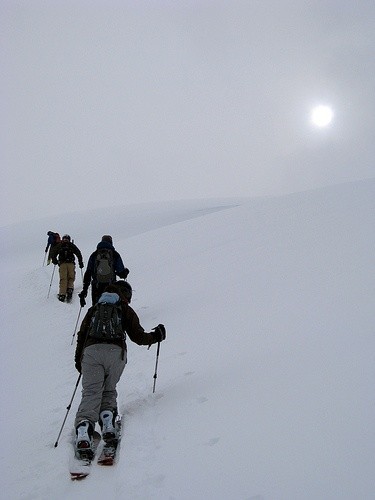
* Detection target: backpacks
[93,248,115,289]
[59,242,75,262]
[52,233,61,244]
[83,301,128,360]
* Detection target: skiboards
[69,429,97,480]
[99,410,127,467]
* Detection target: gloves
[155,323,166,342]
[52,258,57,264]
[45,248,48,253]
[125,268,129,275]
[80,290,88,298]
[79,262,84,268]
[74,361,81,372]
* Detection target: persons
[79,234,129,306]
[74,281,167,462]
[45,231,58,265]
[52,234,84,302]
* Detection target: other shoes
[57,294,65,300]
[99,409,118,443]
[74,419,95,460]
[67,288,73,299]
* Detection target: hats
[115,280,133,304]
[102,235,113,244]
[62,234,71,241]
